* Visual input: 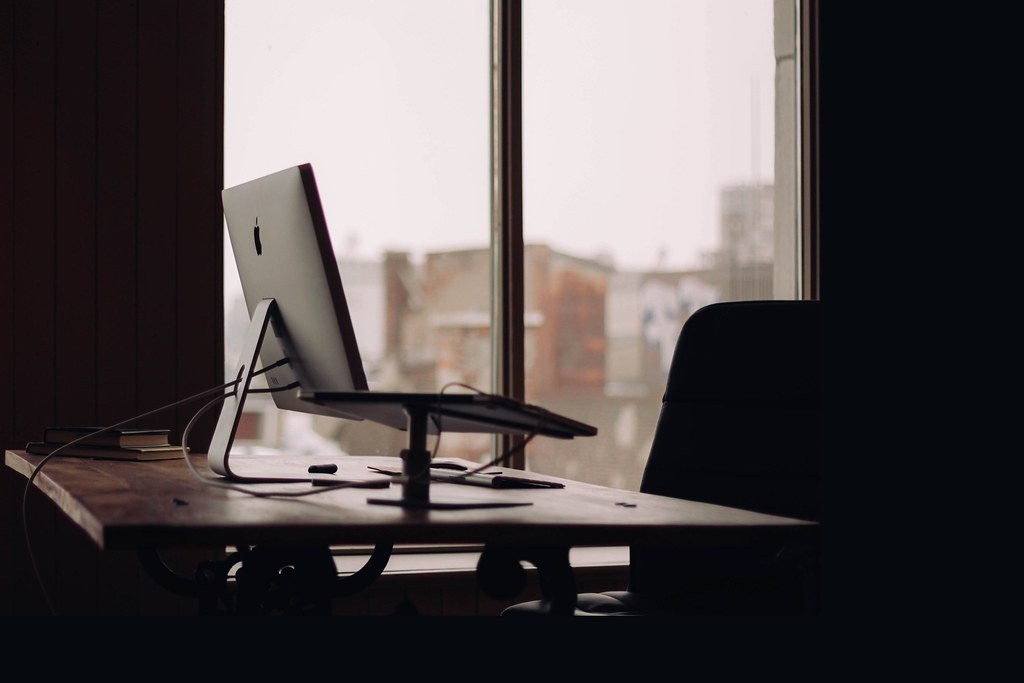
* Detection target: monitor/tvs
[206,162,370,480]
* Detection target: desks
[4,448,822,624]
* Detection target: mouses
[430,460,467,472]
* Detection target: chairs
[499,296,832,619]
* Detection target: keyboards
[368,464,566,491]
